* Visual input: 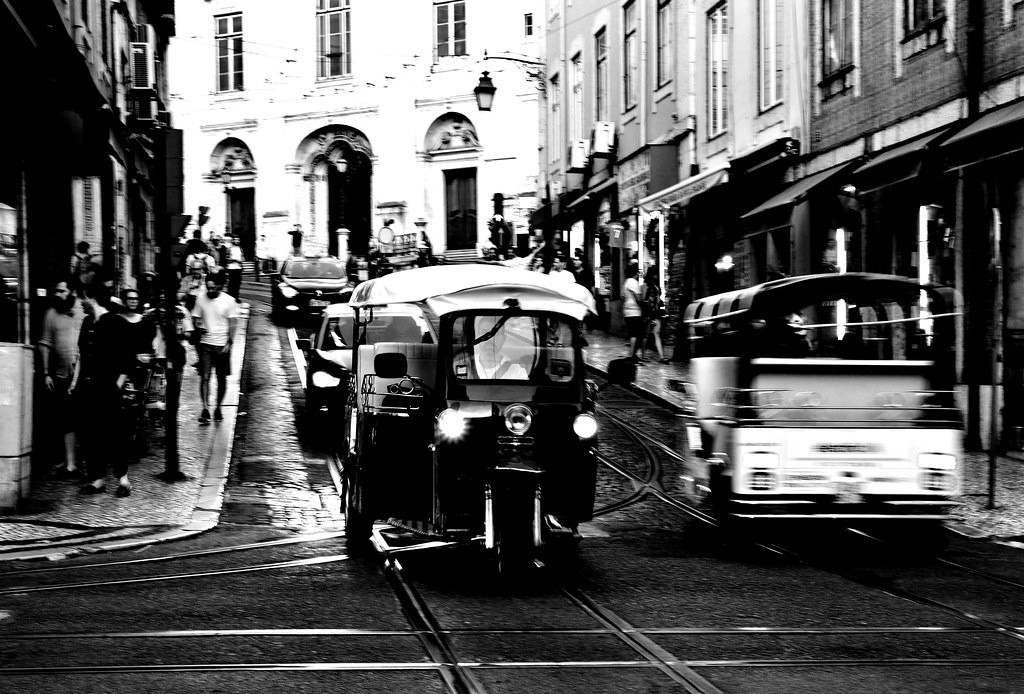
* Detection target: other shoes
[115,485,132,497]
[632,354,670,366]
[213,407,224,422]
[198,409,212,424]
[80,484,106,494]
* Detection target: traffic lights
[199,206,209,225]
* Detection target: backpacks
[74,254,91,274]
[190,254,209,274]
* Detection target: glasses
[127,296,138,300]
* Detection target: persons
[376,317,421,342]
[367,247,382,280]
[290,263,339,276]
[38,230,245,497]
[287,224,304,261]
[621,262,671,364]
[482,239,595,346]
[453,317,529,379]
[253,234,277,282]
[761,308,813,351]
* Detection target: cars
[294,303,437,439]
[269,254,356,324]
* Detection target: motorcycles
[338,263,639,595]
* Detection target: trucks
[668,271,968,557]
